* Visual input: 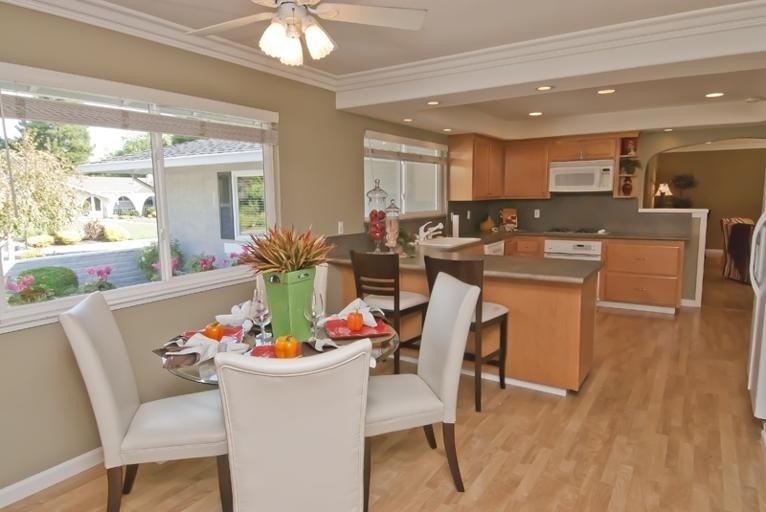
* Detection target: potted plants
[620,159,641,174]
[235,223,336,342]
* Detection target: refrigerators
[745,212,766,421]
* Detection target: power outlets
[338,221,343,234]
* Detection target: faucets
[418,221,444,242]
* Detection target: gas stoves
[547,227,610,236]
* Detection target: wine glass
[250,287,272,340]
[301,293,326,337]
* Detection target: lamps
[259,3,335,67]
[655,183,673,208]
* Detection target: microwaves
[548,159,616,193]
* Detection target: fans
[182,0,428,36]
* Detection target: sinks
[409,236,482,248]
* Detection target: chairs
[214,338,372,512]
[256,262,328,317]
[59,291,233,512]
[350,249,429,375]
[363,271,481,512]
[424,255,510,412]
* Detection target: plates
[324,318,393,340]
[185,326,244,344]
[251,339,304,358]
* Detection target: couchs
[720,218,754,285]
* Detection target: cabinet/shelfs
[516,236,544,256]
[599,239,685,307]
[484,240,505,256]
[549,132,616,161]
[448,133,505,201]
[613,130,643,199]
[504,137,551,199]
[504,236,516,256]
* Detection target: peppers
[348,308,363,331]
[205,321,223,341]
[275,335,298,358]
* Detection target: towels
[301,337,338,357]
[215,300,254,330]
[317,298,389,328]
[162,332,221,369]
